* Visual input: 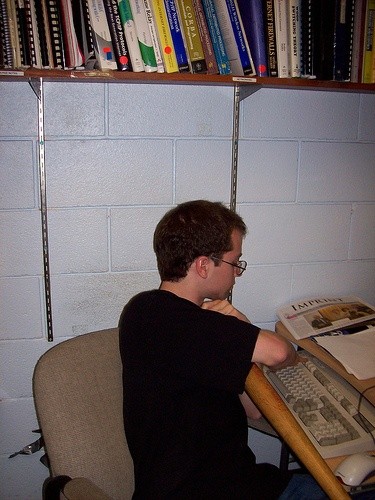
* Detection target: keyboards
[263,349,375,456]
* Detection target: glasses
[206,256,247,276]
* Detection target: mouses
[335,452,375,487]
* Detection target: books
[0,0,374,86]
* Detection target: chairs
[8,328,289,500]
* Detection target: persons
[118,200,330,500]
